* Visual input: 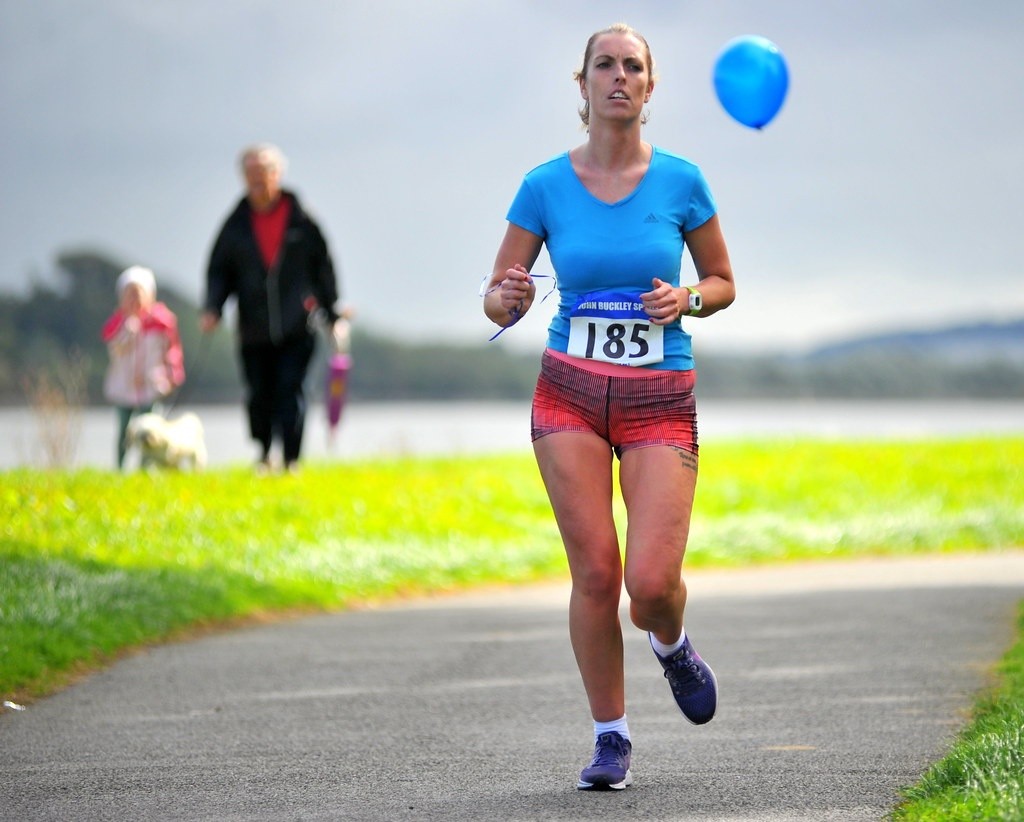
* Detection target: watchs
[686,286,702,317]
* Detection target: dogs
[118,412,209,470]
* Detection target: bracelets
[509,311,527,319]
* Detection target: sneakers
[577,731,632,790]
[648,626,717,726]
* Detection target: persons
[201,144,340,471]
[102,267,185,469]
[482,25,737,792]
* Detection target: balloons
[712,35,790,128]
